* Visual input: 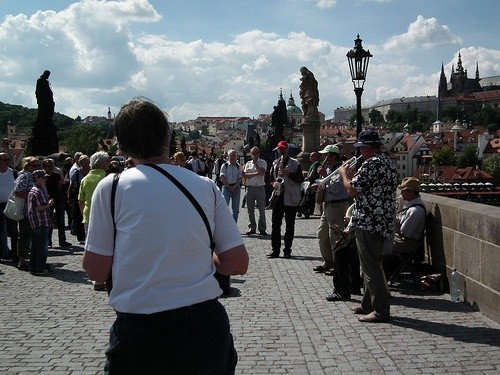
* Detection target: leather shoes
[358,312,390,322]
[350,305,371,313]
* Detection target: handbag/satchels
[3,172,28,220]
[69,169,80,199]
[406,263,445,292]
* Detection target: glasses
[3,158,9,162]
[29,164,41,168]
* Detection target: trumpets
[316,155,363,189]
[396,195,403,210]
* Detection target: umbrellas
[270,143,302,157]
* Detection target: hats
[353,131,383,147]
[399,177,420,191]
[277,141,288,149]
[318,144,339,154]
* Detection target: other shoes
[284,252,291,257]
[246,229,256,234]
[29,267,53,275]
[326,292,351,301]
[325,268,334,275]
[350,289,361,295]
[94,284,106,291]
[61,242,72,249]
[313,266,332,271]
[260,230,267,235]
[297,212,302,217]
[266,252,279,257]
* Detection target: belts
[325,198,349,205]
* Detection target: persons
[337,129,401,322]
[0,136,139,290]
[325,176,428,302]
[313,146,352,274]
[168,145,350,218]
[251,131,261,146]
[263,140,305,258]
[243,146,269,236]
[299,66,320,121]
[271,106,284,137]
[81,98,250,375]
[219,149,243,224]
[34,70,55,117]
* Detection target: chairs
[387,227,430,286]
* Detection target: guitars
[316,156,330,204]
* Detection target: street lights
[345,33,373,158]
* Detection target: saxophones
[276,155,284,196]
